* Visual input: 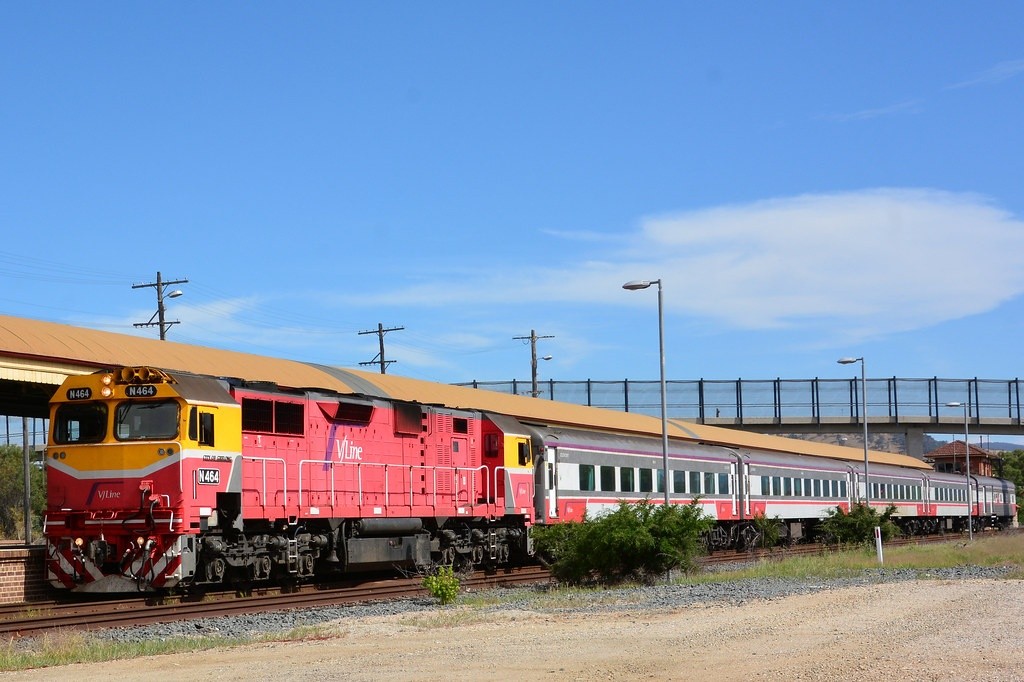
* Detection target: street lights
[947,402,974,542]
[522,355,552,398]
[836,357,870,508]
[623,278,672,587]
[133,289,184,340]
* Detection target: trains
[45,367,1015,600]
